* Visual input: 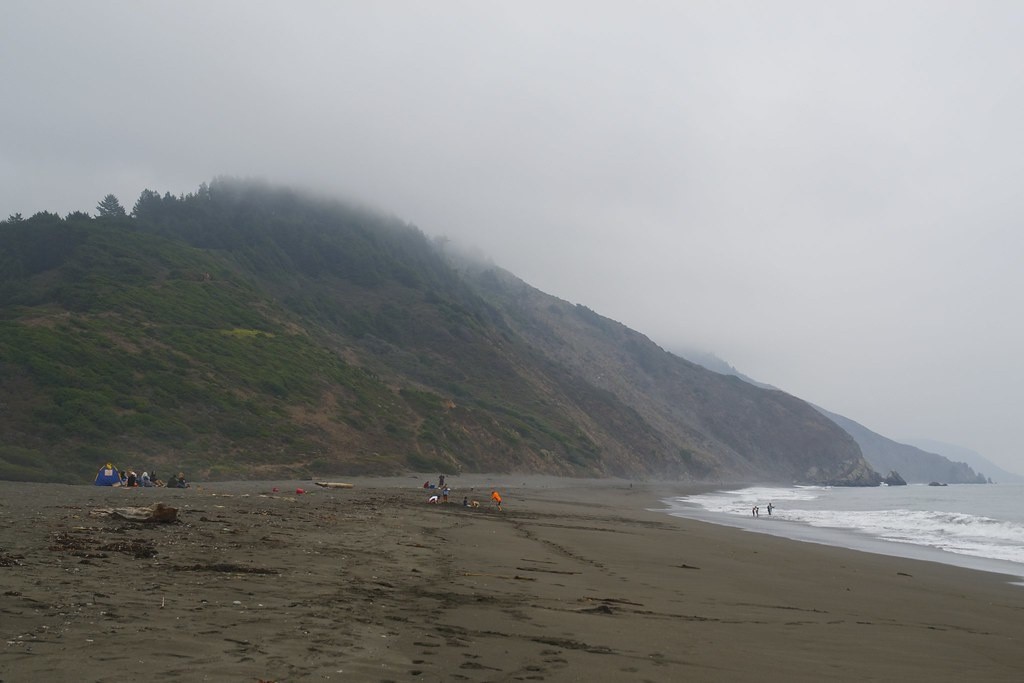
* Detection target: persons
[463,496,480,508]
[490,491,503,512]
[767,503,774,515]
[121,468,191,488]
[753,506,759,517]
[423,474,451,504]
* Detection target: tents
[94,463,123,486]
